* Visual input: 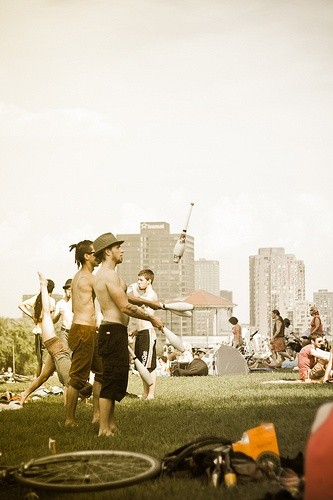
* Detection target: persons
[18,279,55,390]
[229,317,244,349]
[282,318,301,358]
[65,240,103,428]
[298,333,333,384]
[270,309,294,362]
[91,232,165,438]
[20,270,93,406]
[310,306,325,340]
[52,278,87,360]
[299,336,309,347]
[133,269,160,401]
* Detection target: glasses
[315,340,322,343]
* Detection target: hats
[198,348,206,354]
[62,279,72,289]
[93,232,124,257]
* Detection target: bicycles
[0,446,162,492]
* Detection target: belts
[61,327,70,333]
